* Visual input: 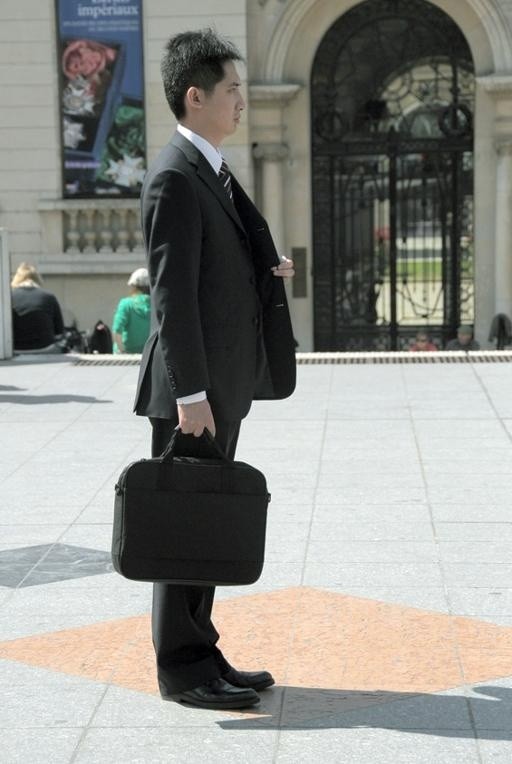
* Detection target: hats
[126,267,151,288]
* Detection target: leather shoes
[156,666,280,709]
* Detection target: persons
[443,323,479,351]
[133,28,299,713]
[9,260,66,356]
[111,267,152,354]
[409,328,437,351]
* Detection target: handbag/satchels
[112,422,276,585]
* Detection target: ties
[214,159,237,210]
